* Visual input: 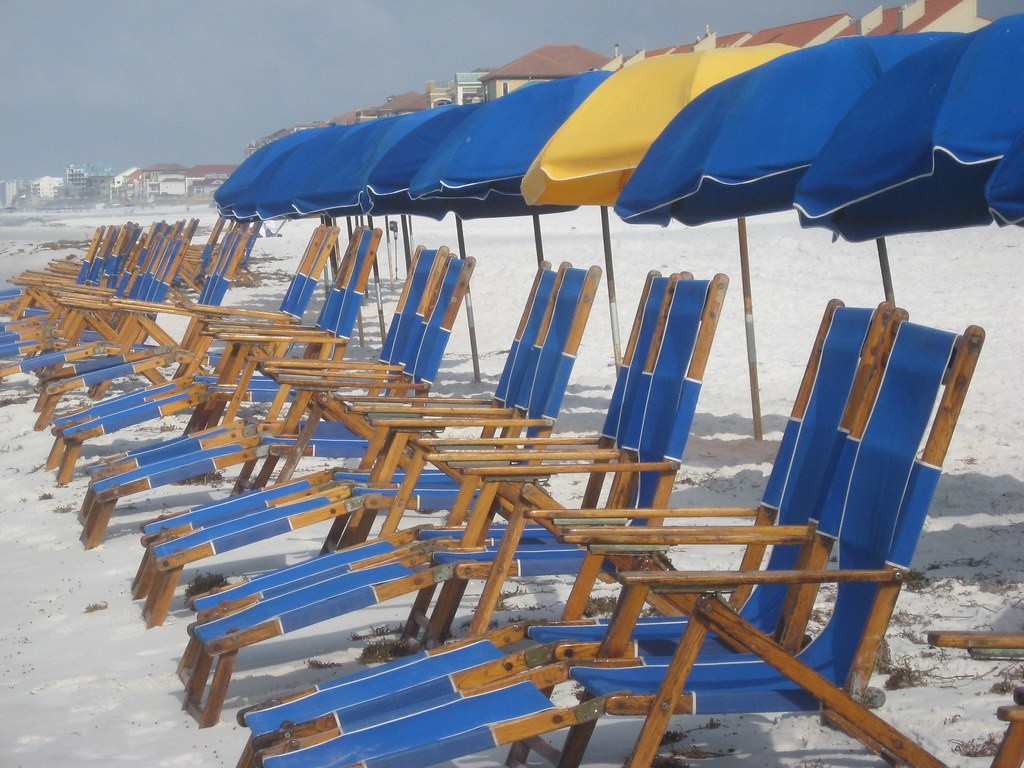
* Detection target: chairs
[0,216,1024,768]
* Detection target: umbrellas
[612,31,967,306]
[408,71,622,379]
[520,44,803,441]
[212,101,579,383]
[984,129,1024,227]
[791,10,1024,244]
